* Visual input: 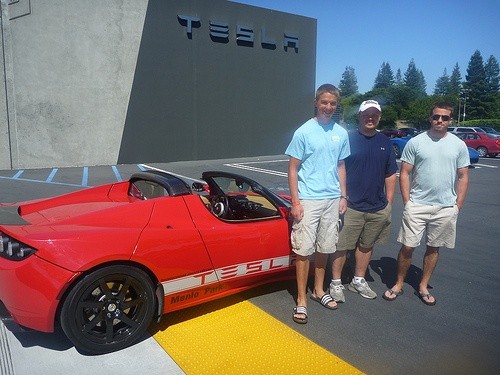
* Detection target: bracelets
[292,201,300,207]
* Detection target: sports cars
[0,169,345,358]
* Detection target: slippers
[414,290,437,306]
[293,306,308,324]
[311,293,337,310]
[384,289,403,301]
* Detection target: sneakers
[330,279,346,303]
[347,278,377,299]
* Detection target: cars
[447,125,500,166]
[381,127,426,159]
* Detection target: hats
[358,100,381,112]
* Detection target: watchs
[341,196,347,199]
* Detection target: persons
[329,100,399,303]
[285,84,351,325]
[383,102,471,305]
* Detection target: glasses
[431,115,450,120]
